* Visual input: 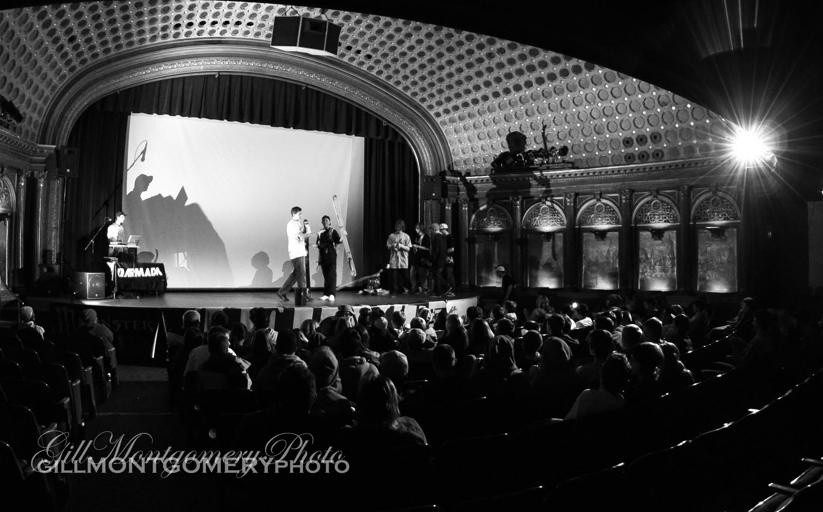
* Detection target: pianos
[109,242,139,247]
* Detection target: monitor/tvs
[127,234,141,244]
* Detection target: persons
[106,211,128,256]
[311,214,346,302]
[276,205,310,301]
[428,222,445,298]
[384,219,411,298]
[410,222,430,296]
[439,222,456,298]
[495,266,514,301]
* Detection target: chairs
[0,308,822,512]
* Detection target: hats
[114,210,127,218]
[134,173,153,186]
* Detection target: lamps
[268,5,341,56]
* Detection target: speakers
[78,272,106,300]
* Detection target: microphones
[106,217,112,222]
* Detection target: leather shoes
[302,295,314,303]
[274,290,290,302]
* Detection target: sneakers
[327,294,335,302]
[318,294,328,302]
